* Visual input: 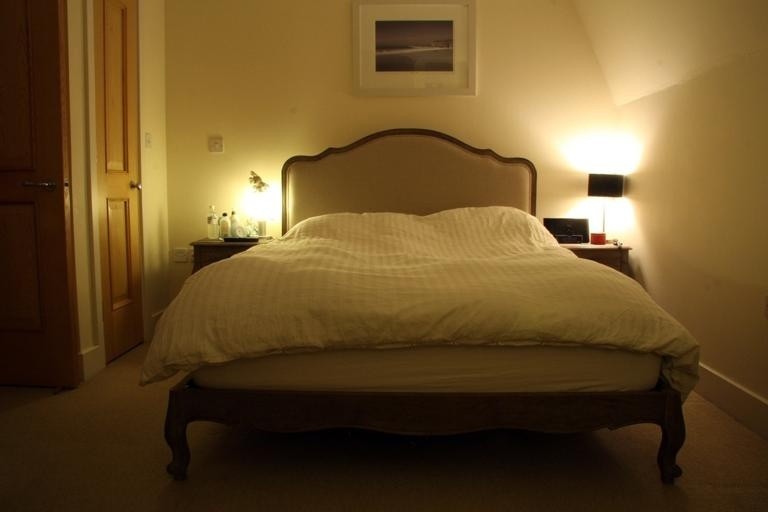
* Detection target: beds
[160,127,689,483]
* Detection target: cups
[591,233,606,245]
[258,221,267,236]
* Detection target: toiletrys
[219,212,230,239]
[228,211,239,236]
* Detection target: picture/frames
[352,0,478,96]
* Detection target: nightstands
[188,236,274,278]
[555,239,633,280]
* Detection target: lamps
[587,170,625,243]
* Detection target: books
[223,233,273,242]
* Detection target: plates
[224,237,259,242]
[555,235,583,243]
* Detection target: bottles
[206,204,239,239]
